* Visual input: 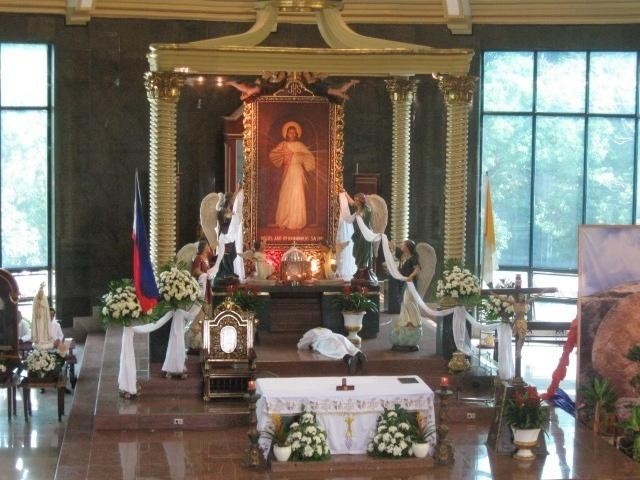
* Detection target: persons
[17,311,31,344]
[379,233,437,328]
[337,184,388,280]
[176,240,211,309]
[296,325,369,376]
[269,121,315,231]
[199,189,243,278]
[32,307,64,350]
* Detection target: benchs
[20,354,77,421]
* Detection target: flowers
[330,286,378,315]
[259,415,291,446]
[96,278,142,327]
[283,409,330,460]
[25,348,63,379]
[494,390,556,440]
[367,403,417,458]
[441,258,481,300]
[483,280,513,321]
[411,412,437,442]
[157,264,203,303]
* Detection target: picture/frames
[243,96,345,255]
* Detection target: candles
[442,377,448,385]
[248,381,256,390]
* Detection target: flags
[480,175,499,289]
[131,170,161,314]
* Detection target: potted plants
[583,377,616,434]
[613,405,640,462]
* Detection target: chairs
[199,296,259,403]
[0,268,19,423]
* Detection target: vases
[273,443,292,462]
[341,310,366,349]
[413,443,429,458]
[509,423,541,461]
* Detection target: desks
[252,374,437,455]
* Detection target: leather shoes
[347,356,360,374]
[357,351,368,370]
[40,388,44,393]
[64,387,70,393]
